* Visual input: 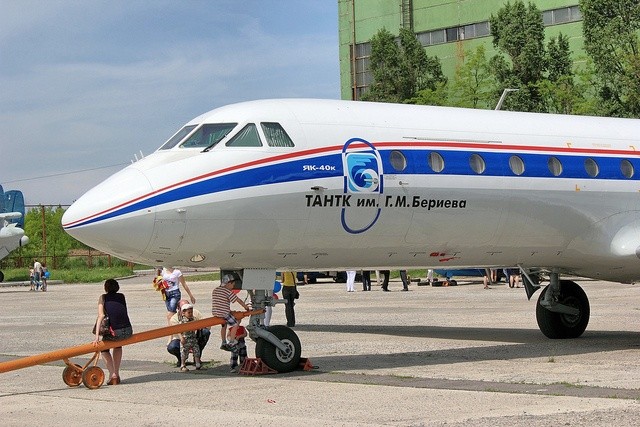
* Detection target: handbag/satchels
[295,290,299,299]
[92,314,111,336]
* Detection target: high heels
[107,376,118,385]
[117,376,120,384]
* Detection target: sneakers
[180,366,189,371]
[220,344,231,351]
[196,366,208,370]
[226,342,239,356]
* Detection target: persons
[380,270,390,291]
[264,294,277,327]
[490,268,497,282]
[154,267,196,345]
[400,270,408,291]
[346,270,357,292]
[90,279,133,385]
[167,300,211,366]
[227,317,247,375]
[279,271,297,328]
[212,274,253,354]
[362,271,371,291]
[177,304,201,371]
[508,269,521,288]
[302,272,309,284]
[29,258,48,292]
[483,271,492,289]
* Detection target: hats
[220,274,235,286]
[181,304,193,312]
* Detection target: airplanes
[0,212,30,283]
[60,98,640,375]
